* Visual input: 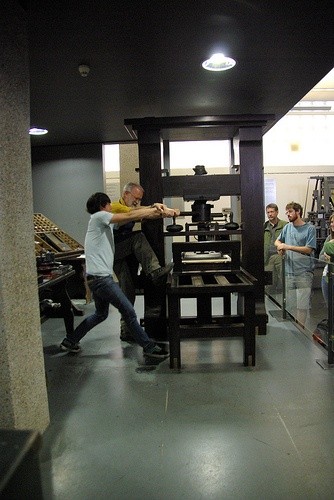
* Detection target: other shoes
[60,338,81,352]
[143,344,171,358]
[120,333,133,341]
[151,262,174,283]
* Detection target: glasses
[285,209,293,215]
[129,191,143,202]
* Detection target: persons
[264,203,290,293]
[273,202,334,328]
[60,196,173,359]
[107,183,180,342]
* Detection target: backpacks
[313,318,329,347]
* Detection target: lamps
[202,53,237,71]
